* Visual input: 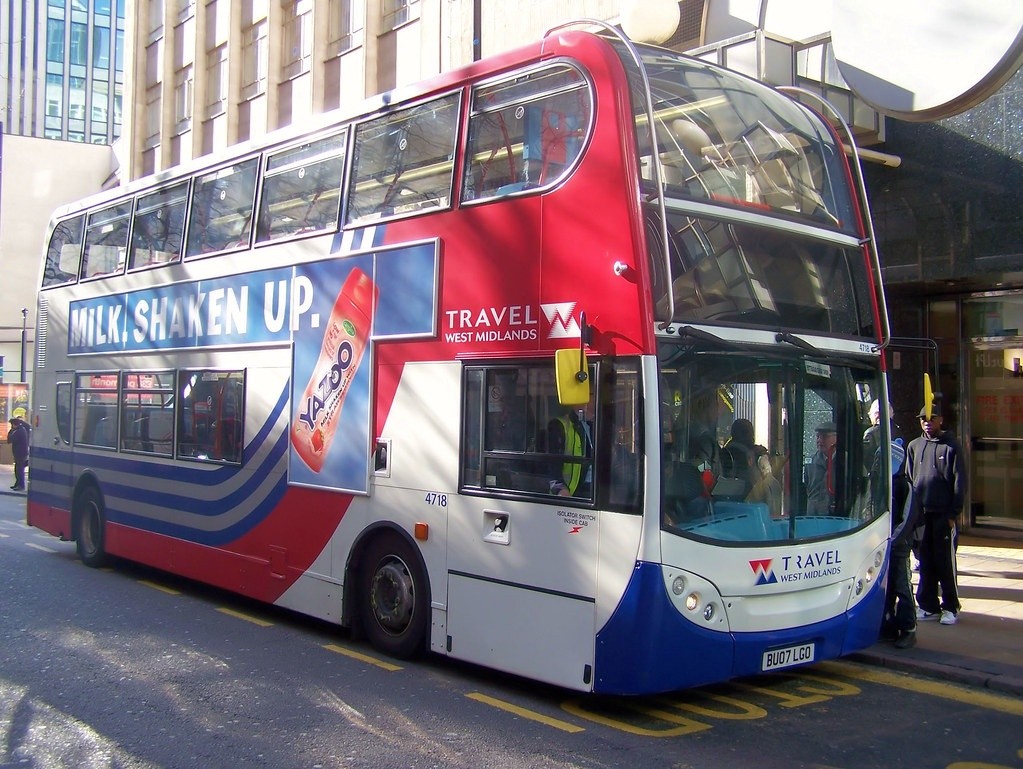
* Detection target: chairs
[92,402,240,466]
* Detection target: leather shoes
[879,624,899,640]
[895,632,918,648]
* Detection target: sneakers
[940,607,962,625]
[915,607,943,620]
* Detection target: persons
[540,381,594,496]
[807,403,969,646]
[674,419,771,521]
[6,417,30,491]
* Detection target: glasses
[815,433,837,439]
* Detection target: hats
[871,438,905,475]
[916,404,940,417]
[815,421,837,431]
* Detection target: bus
[27,18,894,705]
